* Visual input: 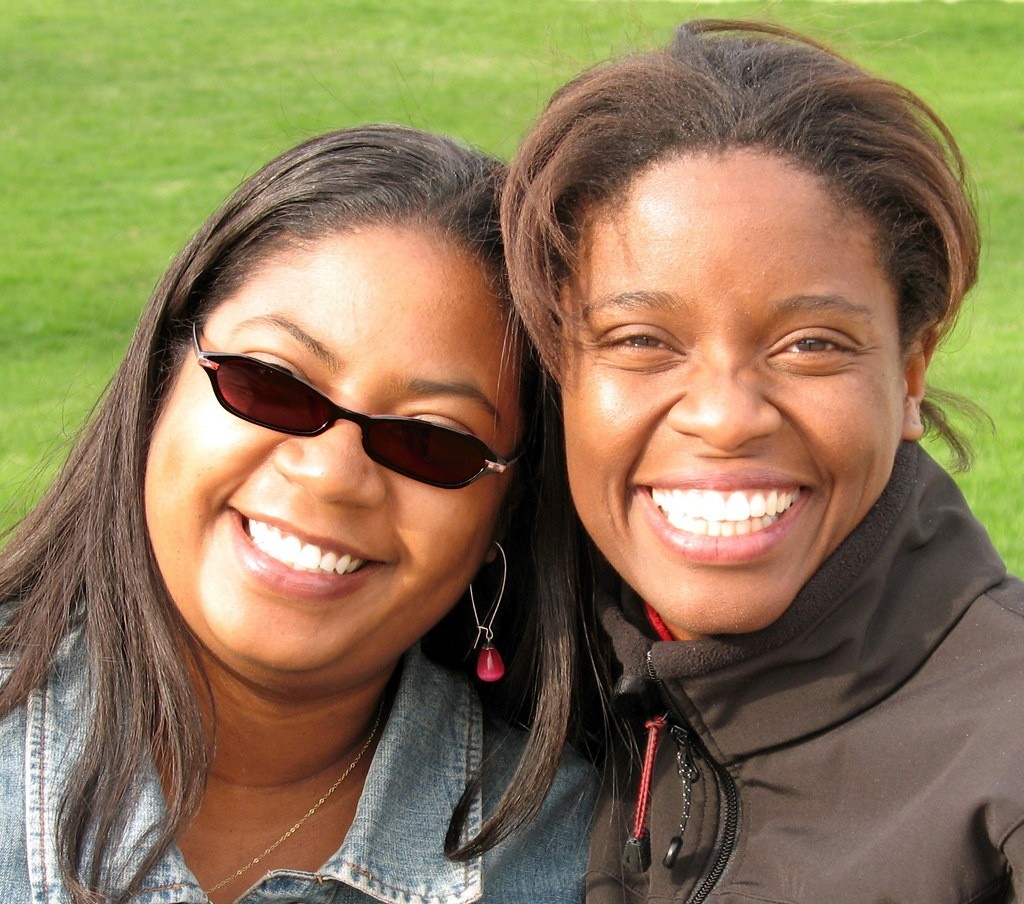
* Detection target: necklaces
[201,695,386,894]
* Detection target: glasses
[192,321,525,489]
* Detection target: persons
[0,119,604,904]
[499,22,1024,904]
